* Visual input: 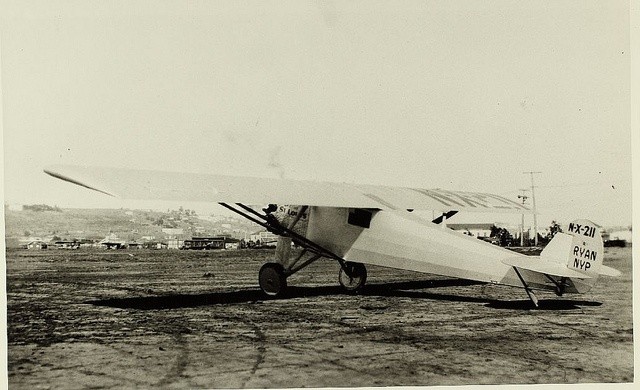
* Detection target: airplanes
[42,167,629,307]
